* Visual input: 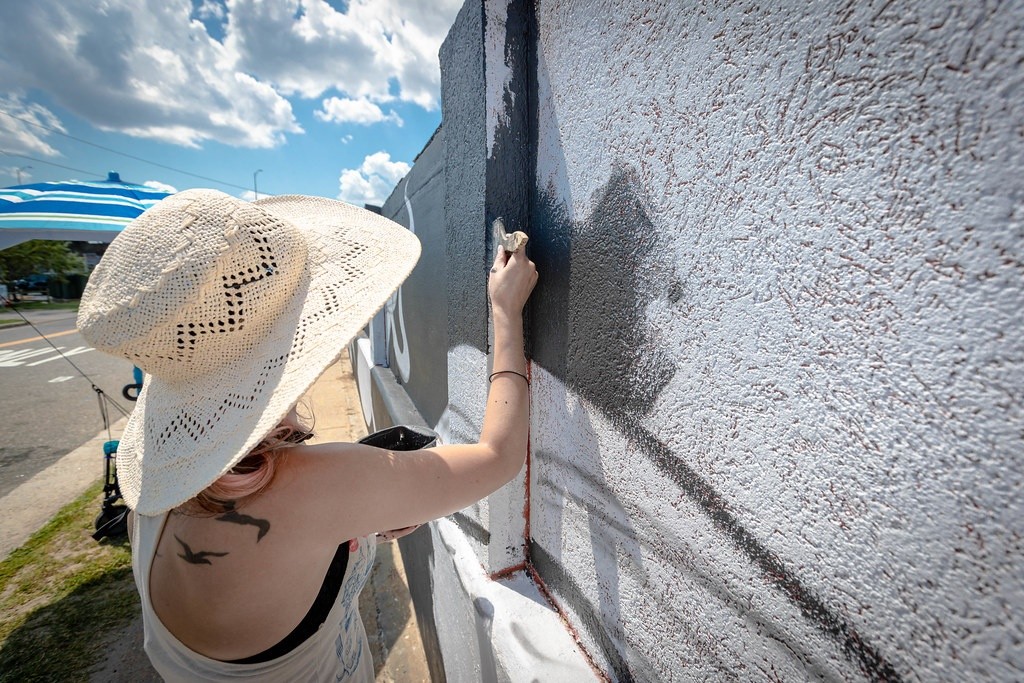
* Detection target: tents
[0,171,173,251]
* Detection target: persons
[75,188,539,683]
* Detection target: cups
[354,425,440,451]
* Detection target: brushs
[489,216,528,263]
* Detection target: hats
[76,187,423,516]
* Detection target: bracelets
[489,370,529,388]
